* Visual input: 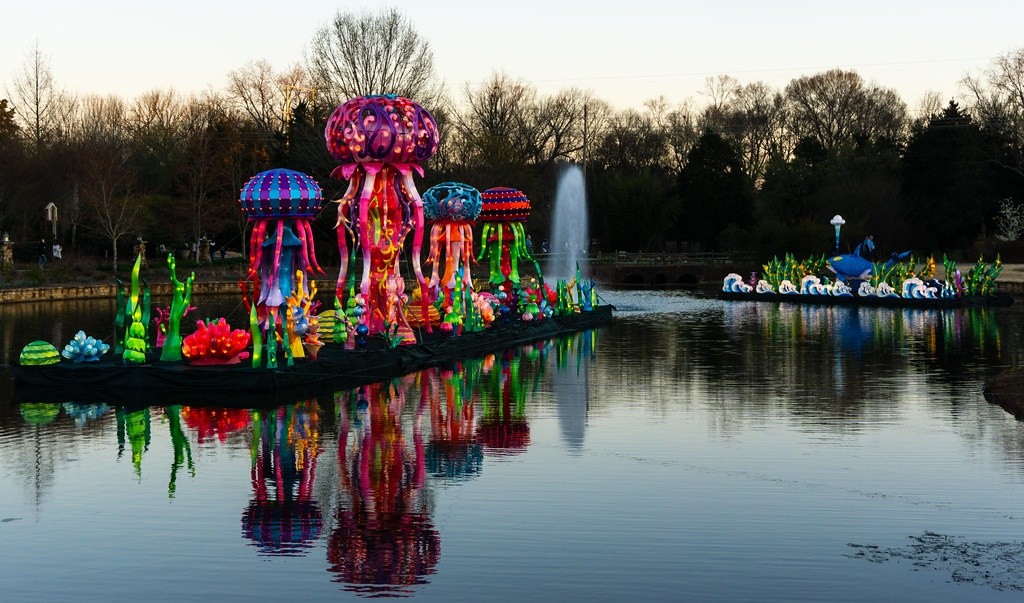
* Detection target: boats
[11,304,616,410]
[712,291,1015,310]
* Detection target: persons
[38,239,47,269]
[209,239,216,263]
[526,235,532,253]
[541,240,548,259]
[52,240,62,261]
[220,247,226,258]
[862,234,880,262]
